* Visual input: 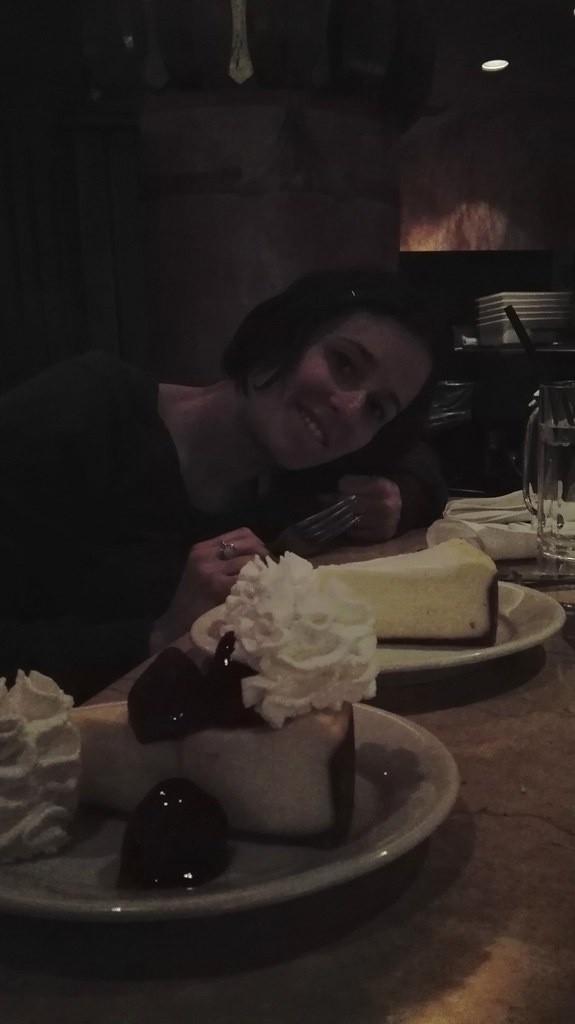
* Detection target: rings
[218,539,238,560]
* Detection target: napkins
[426,484,575,562]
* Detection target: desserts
[0,612,380,894]
[217,536,499,644]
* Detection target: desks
[0,533,575,1024]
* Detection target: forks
[267,496,363,552]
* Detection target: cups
[520,376,575,584]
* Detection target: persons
[0,267,449,702]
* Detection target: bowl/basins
[473,290,573,345]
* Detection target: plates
[189,579,567,685]
[0,697,461,920]
